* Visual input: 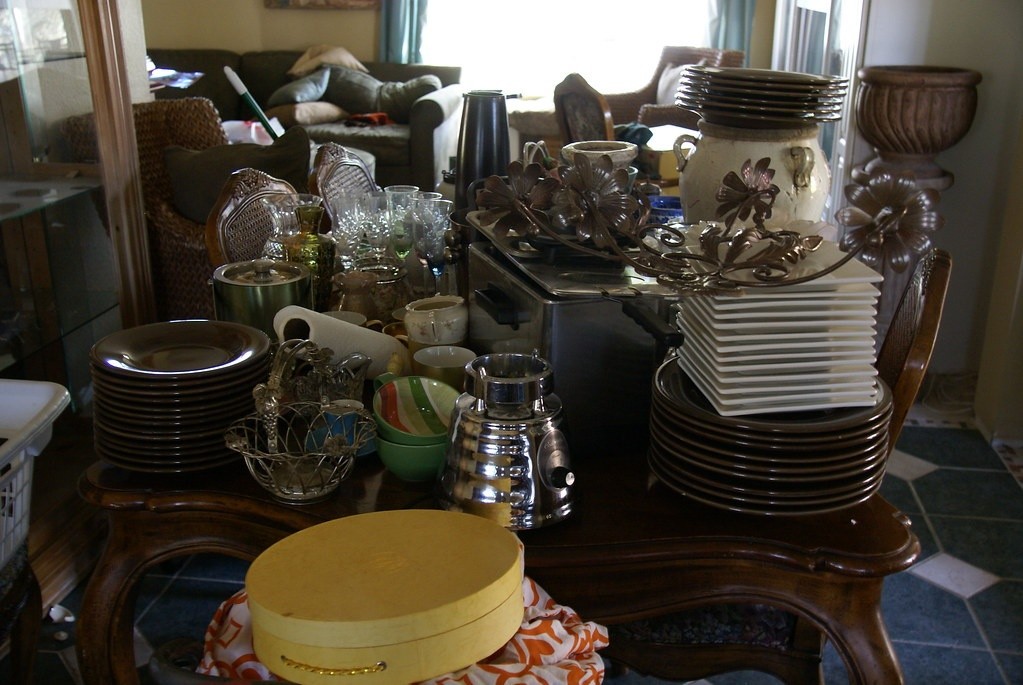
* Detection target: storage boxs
[243,509,524,685]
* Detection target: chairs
[47,96,234,322]
[0,539,44,685]
[204,167,305,267]
[309,142,380,232]
[555,73,615,145]
[597,245,953,685]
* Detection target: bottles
[451,88,511,226]
[259,193,338,313]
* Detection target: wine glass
[331,185,455,296]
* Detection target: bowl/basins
[637,196,684,223]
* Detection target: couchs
[146,47,463,192]
[603,45,744,127]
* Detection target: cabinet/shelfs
[0,0,160,661]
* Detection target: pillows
[657,57,709,105]
[251,44,443,126]
[164,127,309,225]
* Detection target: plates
[648,356,896,517]
[305,426,376,458]
[675,249,887,417]
[88,320,275,477]
[672,63,851,130]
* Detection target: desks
[73,182,920,685]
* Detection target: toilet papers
[273,305,414,381]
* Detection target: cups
[373,372,461,482]
[323,399,364,446]
[321,294,477,395]
